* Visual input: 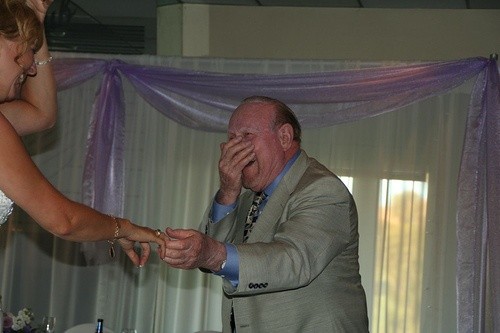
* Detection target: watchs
[220,258,227,272]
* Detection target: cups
[41,316,57,333]
[123,328,137,333]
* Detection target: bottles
[95,318,103,333]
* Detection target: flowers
[4,307,45,333]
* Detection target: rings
[156,229,160,237]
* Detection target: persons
[0,0,170,333]
[158,94,371,333]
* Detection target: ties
[242,192,268,244]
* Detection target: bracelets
[108,214,119,258]
[35,55,55,66]
